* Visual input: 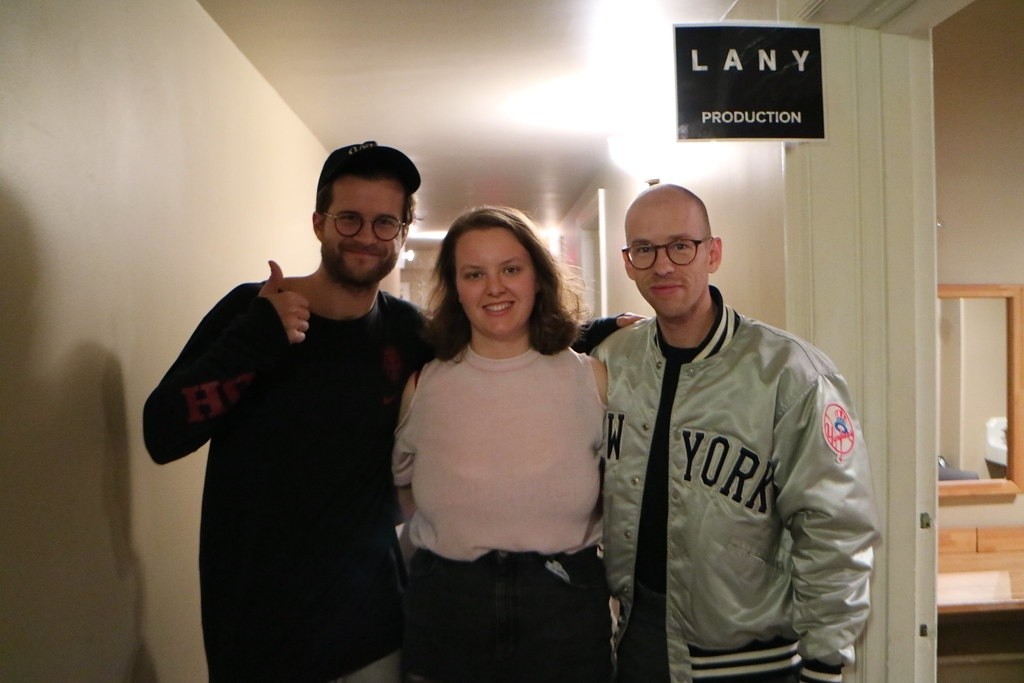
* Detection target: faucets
[939,456,949,469]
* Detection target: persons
[589,184,882,683]
[391,204,609,683]
[142,140,646,683]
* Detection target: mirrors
[935,284,1023,498]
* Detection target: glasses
[322,211,406,241]
[622,237,714,270]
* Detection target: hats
[318,141,421,196]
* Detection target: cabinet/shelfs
[937,525,1024,613]
